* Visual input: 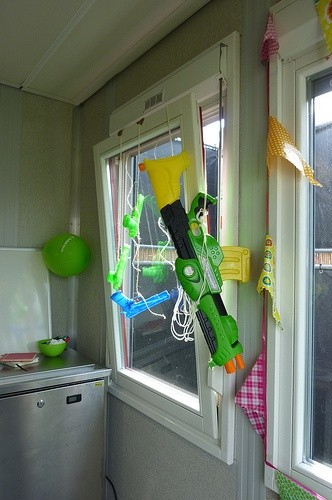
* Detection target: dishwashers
[0,347,112,500]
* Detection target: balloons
[41,232,91,279]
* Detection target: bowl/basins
[39,339,67,357]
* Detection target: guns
[139,150,252,374]
